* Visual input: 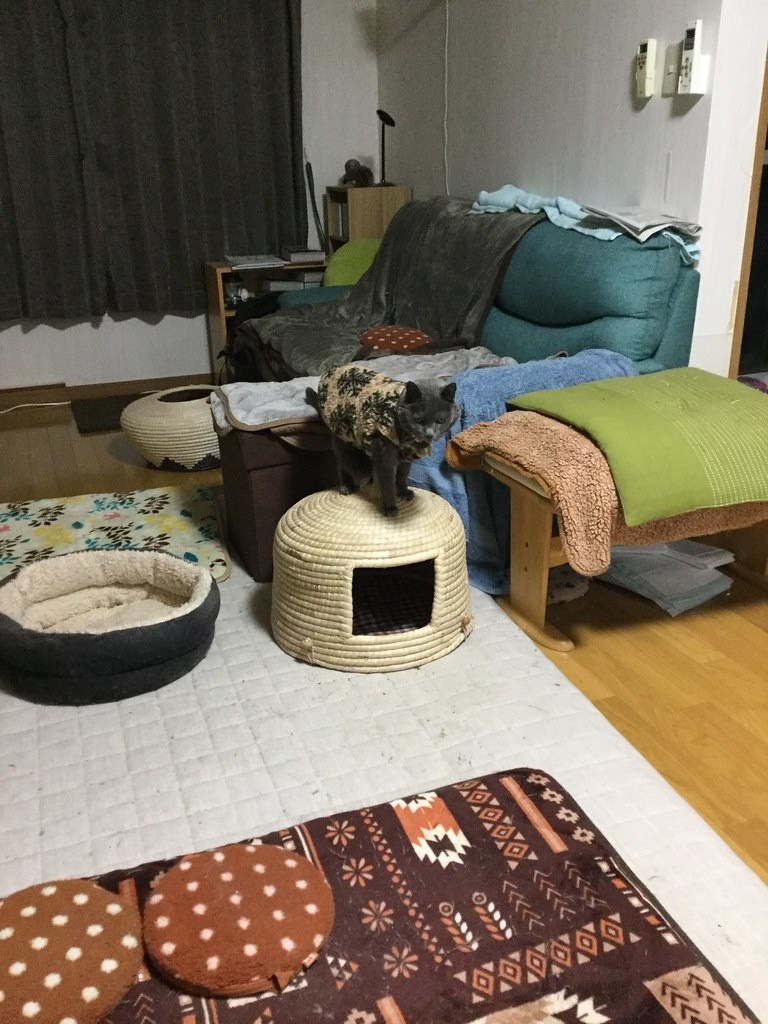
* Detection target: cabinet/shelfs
[207,250,328,386]
[325,182,411,259]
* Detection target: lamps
[375,107,397,187]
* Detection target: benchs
[442,365,768,649]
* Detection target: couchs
[211,194,702,558]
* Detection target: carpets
[0,765,764,1024]
[0,482,231,586]
[0,481,767,1022]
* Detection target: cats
[303,365,460,517]
